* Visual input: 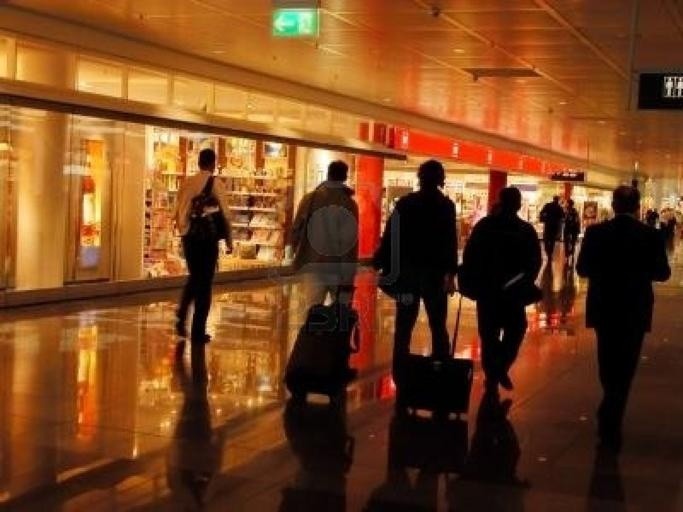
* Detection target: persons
[445,392,532,511]
[535,193,682,274]
[284,159,360,324]
[275,395,349,511]
[170,146,235,340]
[557,255,577,323]
[538,258,557,326]
[584,412,624,511]
[576,182,672,424]
[366,398,449,512]
[454,184,544,391]
[369,156,459,383]
[170,337,228,510]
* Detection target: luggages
[396,291,474,412]
[283,286,355,401]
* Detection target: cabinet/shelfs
[146,170,285,269]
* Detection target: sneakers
[498,370,514,391]
[175,318,186,336]
[191,330,211,339]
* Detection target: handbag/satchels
[189,194,227,242]
[289,227,307,275]
